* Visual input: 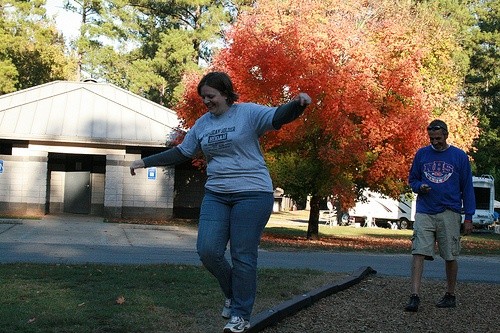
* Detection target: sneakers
[223,316,250,333]
[222,298,232,319]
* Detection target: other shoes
[436,292,457,308]
[405,294,421,312]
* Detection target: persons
[405,119,476,310]
[130,71,312,333]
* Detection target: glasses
[427,125,446,131]
